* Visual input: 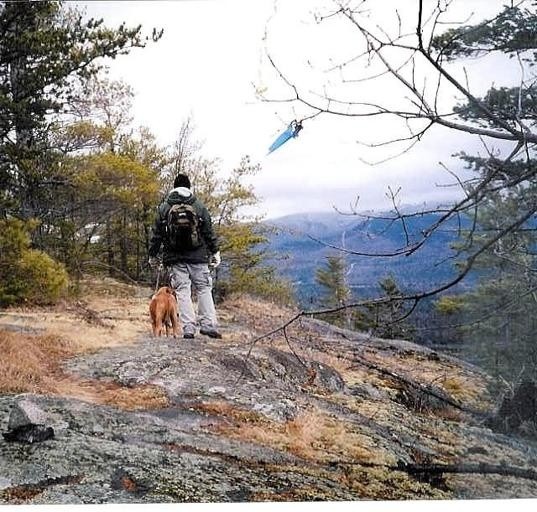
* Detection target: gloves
[207,250,221,270]
[148,256,162,269]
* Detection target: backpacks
[164,200,202,252]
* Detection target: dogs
[148,285,181,341]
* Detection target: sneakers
[182,332,194,339]
[200,326,222,339]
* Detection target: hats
[173,173,191,189]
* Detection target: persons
[146,172,223,340]
